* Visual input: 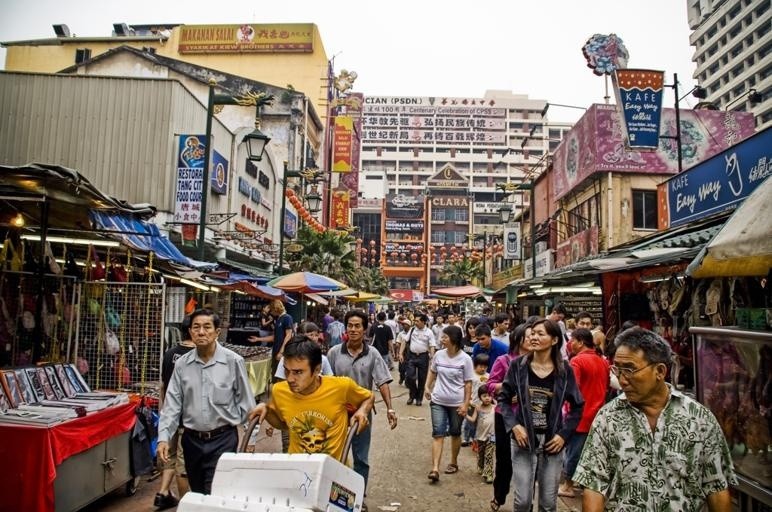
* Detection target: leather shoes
[557,487,576,498]
[416,399,421,406]
[407,397,413,405]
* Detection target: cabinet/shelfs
[232,295,276,329]
[557,297,603,325]
[0,397,142,512]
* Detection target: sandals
[444,464,458,474]
[428,470,439,481]
[490,497,500,511]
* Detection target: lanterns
[355,238,503,265]
[285,188,327,234]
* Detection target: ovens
[688,325,771,508]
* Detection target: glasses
[610,362,653,379]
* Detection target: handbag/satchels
[109,256,127,281]
[402,341,411,360]
[93,314,120,355]
[70,356,89,375]
[85,282,108,298]
[88,246,104,281]
[114,358,130,385]
[43,240,61,273]
[87,300,101,314]
[1,239,22,272]
[106,290,121,327]
[62,286,76,322]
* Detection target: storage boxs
[176,491,314,512]
[210,452,365,512]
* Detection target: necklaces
[182,340,196,347]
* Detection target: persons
[369,312,396,365]
[152,312,193,509]
[561,312,599,429]
[432,313,467,348]
[462,318,483,356]
[327,312,346,347]
[552,329,611,498]
[384,313,396,370]
[324,309,398,512]
[570,325,740,511]
[396,304,466,332]
[423,325,477,482]
[494,317,586,511]
[322,309,338,335]
[490,313,513,347]
[259,303,274,346]
[398,313,437,407]
[456,383,497,484]
[398,319,414,384]
[485,322,538,512]
[249,334,376,477]
[154,308,258,497]
[263,319,337,452]
[246,299,293,390]
[547,305,573,359]
[472,324,511,376]
[458,352,490,448]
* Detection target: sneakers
[399,379,403,384]
[477,467,483,474]
[154,489,179,508]
[483,474,493,483]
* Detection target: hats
[398,318,411,326]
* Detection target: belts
[186,423,231,440]
[411,351,427,356]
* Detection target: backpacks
[694,281,709,325]
[667,278,692,319]
[705,278,728,325]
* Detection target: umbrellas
[369,294,394,303]
[266,271,348,322]
[348,289,382,301]
[310,282,358,305]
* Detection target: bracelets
[387,409,395,414]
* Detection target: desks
[242,357,272,402]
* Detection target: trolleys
[236,413,360,464]
